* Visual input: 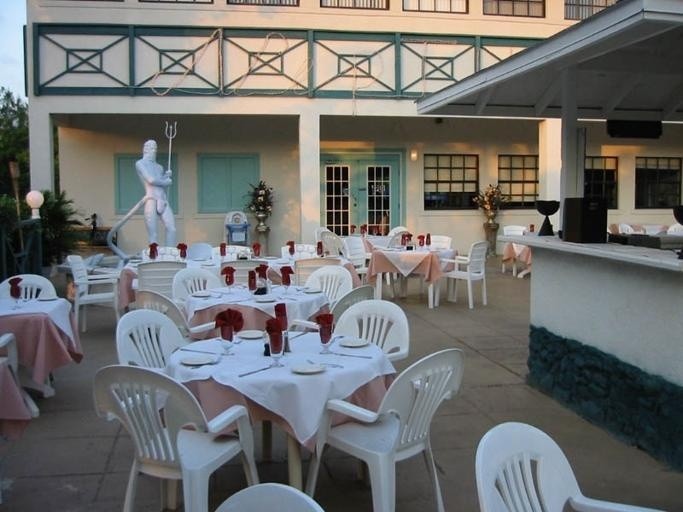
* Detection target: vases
[483,208,499,223]
[255,211,269,228]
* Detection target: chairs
[203,482,324,512]
[206,244,251,262]
[276,243,321,265]
[285,283,374,336]
[473,421,668,512]
[313,227,332,244]
[217,259,269,290]
[292,258,340,283]
[63,254,121,331]
[0,275,56,304]
[92,368,260,512]
[319,231,374,286]
[376,227,412,300]
[361,238,402,298]
[498,226,528,277]
[420,236,452,302]
[434,241,490,311]
[307,348,467,512]
[130,288,219,344]
[330,299,410,369]
[0,333,44,424]
[172,269,223,311]
[372,231,412,299]
[300,264,351,312]
[112,309,189,393]
[140,246,178,264]
[125,259,186,305]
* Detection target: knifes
[290,330,307,340]
[238,367,271,378]
[181,348,221,354]
[340,354,372,359]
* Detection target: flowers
[243,178,275,215]
[469,183,511,212]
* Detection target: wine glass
[349,226,378,237]
[10,287,23,310]
[248,278,256,295]
[277,316,288,336]
[269,334,284,367]
[288,246,323,258]
[282,274,290,297]
[221,326,235,355]
[221,246,260,258]
[226,274,234,294]
[150,247,187,261]
[319,324,332,354]
[260,272,267,283]
[402,238,431,250]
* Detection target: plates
[192,292,211,297]
[182,353,216,366]
[255,297,275,303]
[38,297,58,301]
[305,287,322,293]
[292,363,325,374]
[277,259,289,263]
[193,258,204,261]
[382,244,446,254]
[237,329,265,339]
[202,262,214,266]
[265,256,276,258]
[340,336,369,347]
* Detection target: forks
[307,360,344,369]
